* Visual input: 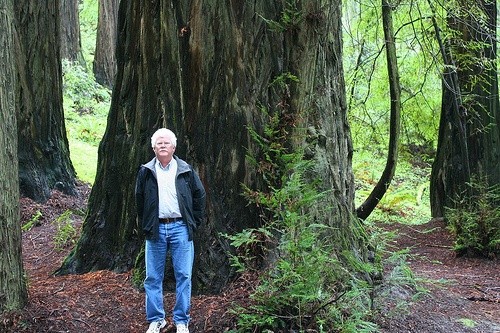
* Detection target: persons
[135,128,206,333]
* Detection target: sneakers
[146,319,166,333]
[176,323,189,333]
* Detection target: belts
[159,217,183,224]
